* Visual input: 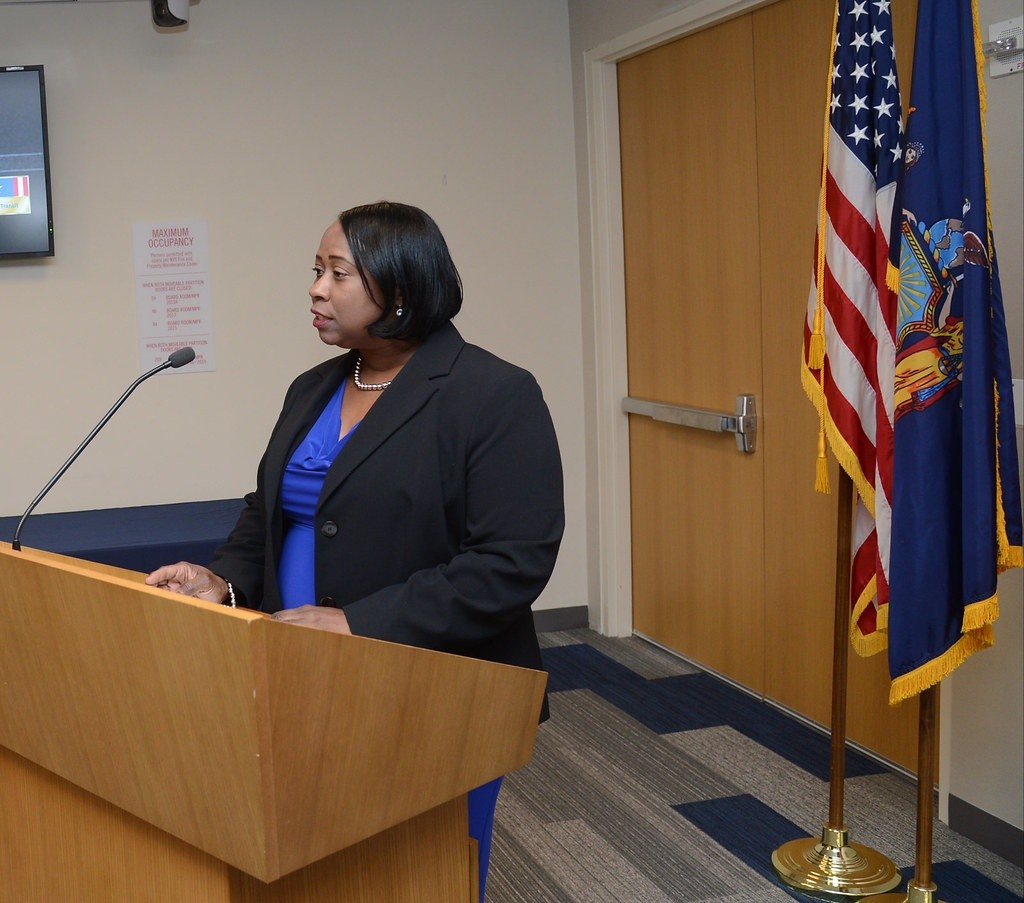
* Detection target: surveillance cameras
[152,0,189,27]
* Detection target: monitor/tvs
[0,64,56,259]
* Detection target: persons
[144,201,565,903]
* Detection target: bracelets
[222,578,236,608]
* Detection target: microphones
[13,347,196,551]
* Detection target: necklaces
[355,357,391,391]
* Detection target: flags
[884,0,1024,706]
[801,0,903,657]
